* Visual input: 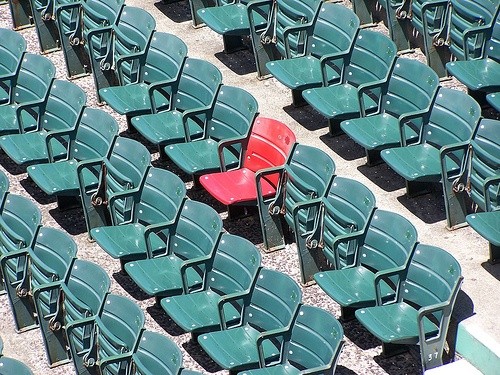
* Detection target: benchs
[0,0,500,375]
